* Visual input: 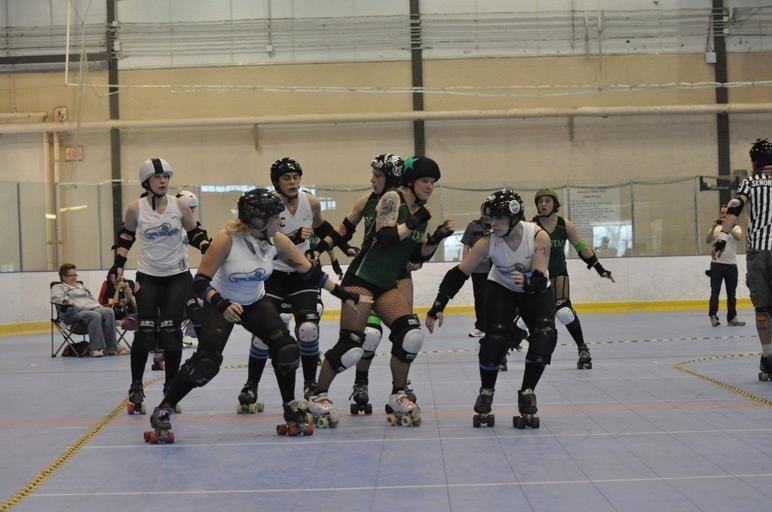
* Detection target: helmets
[480,189,526,222]
[534,185,560,213]
[750,139,772,167]
[238,188,286,226]
[271,156,303,186]
[139,158,174,186]
[370,152,404,187]
[176,189,202,209]
[402,155,441,187]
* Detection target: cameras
[717,219,723,224]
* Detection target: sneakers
[91,343,120,359]
[710,311,723,328]
[468,326,484,339]
[729,317,746,326]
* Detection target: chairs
[99,278,134,349]
[49,279,95,356]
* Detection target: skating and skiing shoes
[498,355,512,372]
[473,383,496,428]
[126,382,147,417]
[151,349,167,372]
[759,353,772,380]
[576,343,594,370]
[304,381,339,430]
[235,380,266,415]
[386,381,424,427]
[350,381,373,418]
[513,388,540,430]
[145,383,183,445]
[276,400,311,439]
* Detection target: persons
[706,202,749,328]
[426,186,558,429]
[143,153,456,444]
[102,156,210,415]
[461,202,492,337]
[49,263,131,359]
[710,143,772,384]
[530,189,613,369]
